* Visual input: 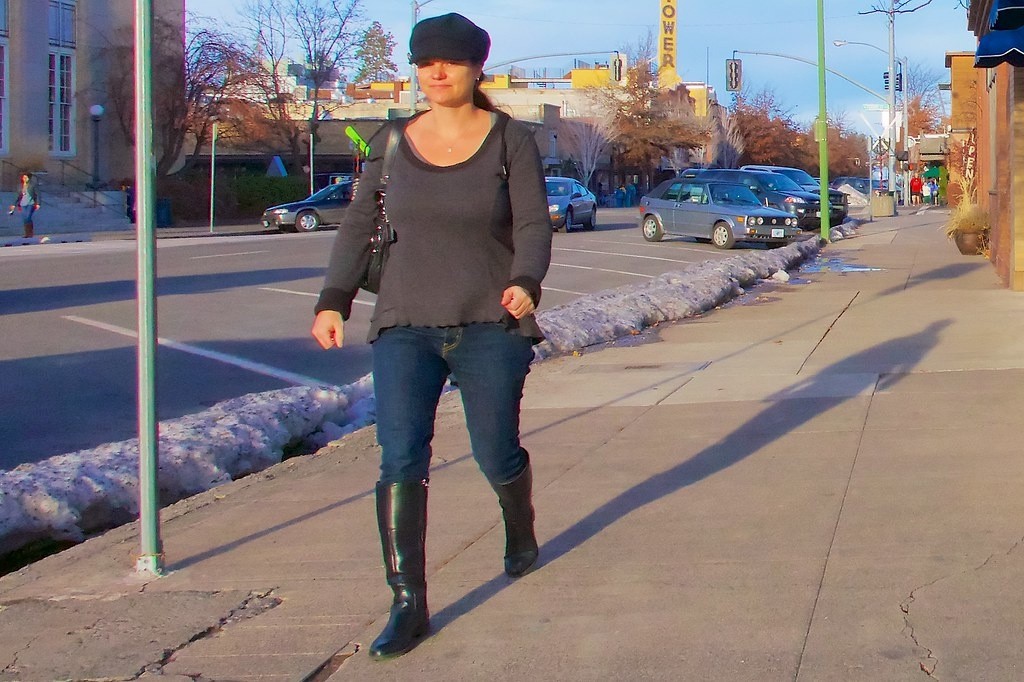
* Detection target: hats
[409,12,491,65]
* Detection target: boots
[22,221,33,238]
[490,446,539,578]
[369,479,430,661]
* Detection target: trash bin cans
[873,191,895,217]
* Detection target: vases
[953,226,983,256]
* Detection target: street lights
[90,105,104,180]
[833,40,909,205]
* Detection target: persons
[625,182,636,205]
[910,173,937,206]
[311,13,554,662]
[11,172,40,237]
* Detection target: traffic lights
[883,72,902,91]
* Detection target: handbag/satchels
[360,116,409,295]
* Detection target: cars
[639,178,802,250]
[262,182,353,232]
[741,165,849,227]
[833,177,902,203]
[680,169,832,231]
[545,176,597,233]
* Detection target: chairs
[690,188,703,202]
